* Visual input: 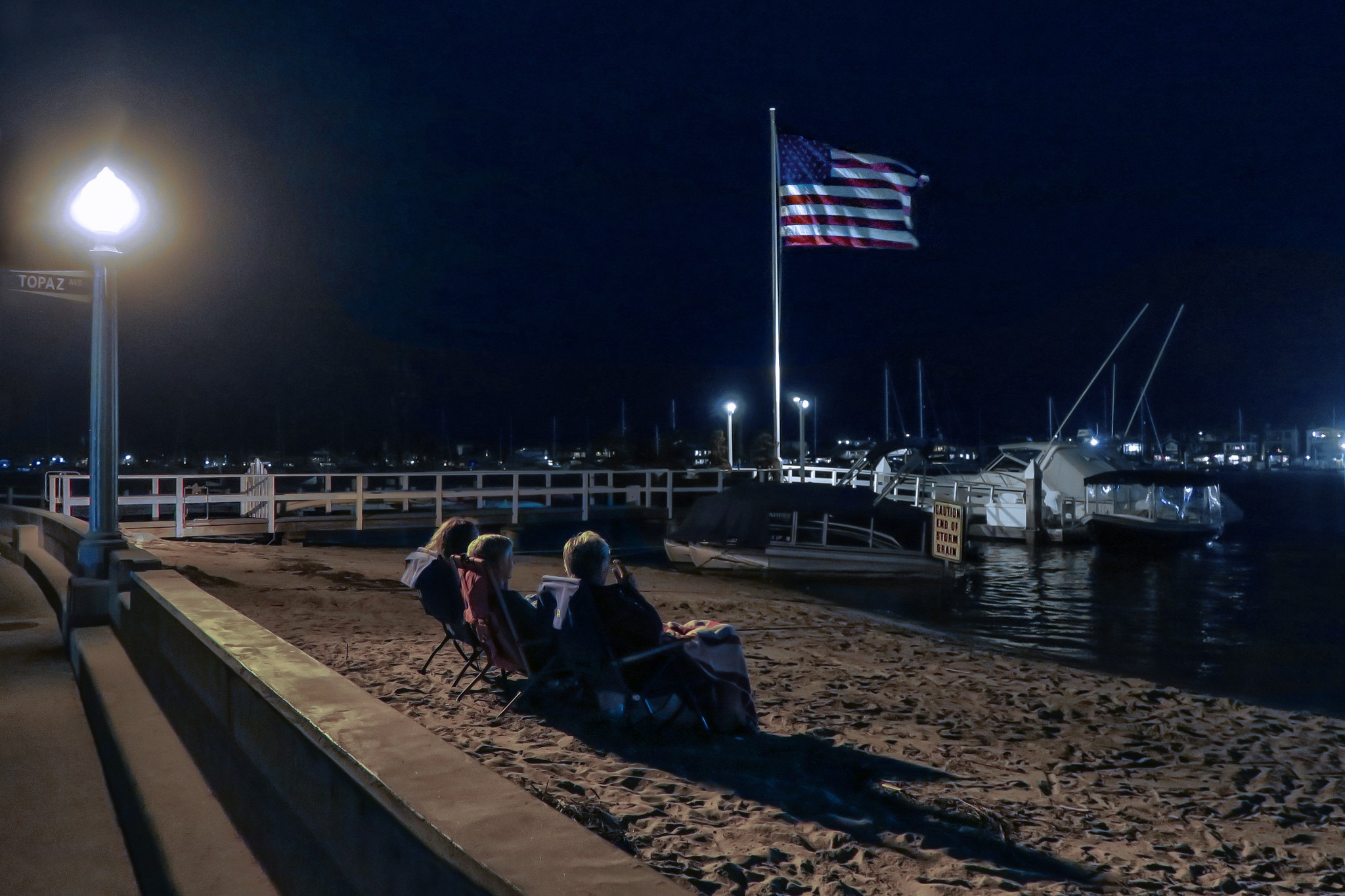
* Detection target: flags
[776,132,932,252]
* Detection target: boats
[873,293,1250,545]
[662,508,975,581]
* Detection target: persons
[467,533,562,675]
[562,531,758,735]
[426,515,479,560]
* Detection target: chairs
[417,547,709,732]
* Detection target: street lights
[725,402,736,468]
[792,396,809,483]
[68,166,142,583]
